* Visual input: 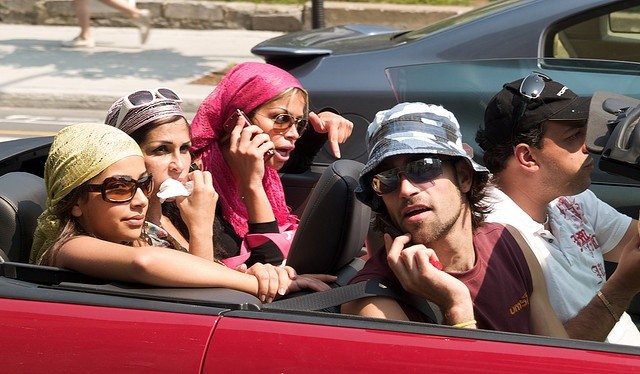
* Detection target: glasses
[510,71,552,152]
[255,111,310,135]
[369,155,444,195]
[80,173,156,203]
[115,87,184,129]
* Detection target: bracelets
[595,289,620,323]
[451,319,477,330]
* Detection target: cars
[251,1,640,211]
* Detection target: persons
[29,122,339,304]
[341,100,571,340]
[190,62,355,268]
[61,1,154,47]
[104,86,225,272]
[470,76,640,346]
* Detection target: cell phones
[222,108,274,163]
[376,212,414,251]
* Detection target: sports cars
[0,135,640,374]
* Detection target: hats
[354,101,491,214]
[476,78,592,152]
